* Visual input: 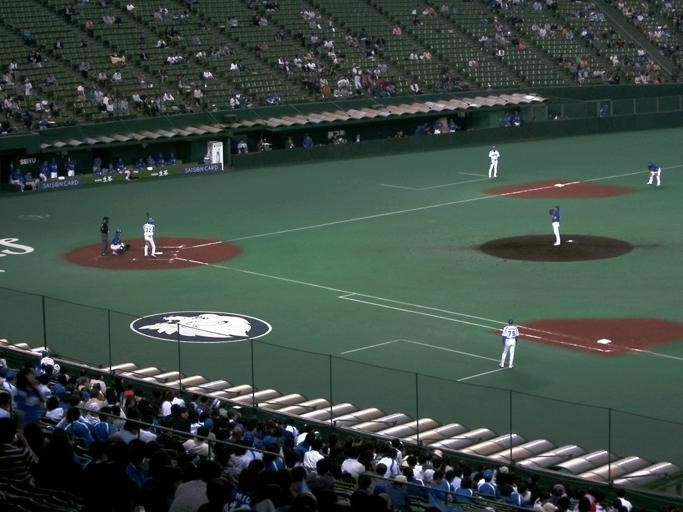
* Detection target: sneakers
[101,250,156,258]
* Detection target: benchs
[335,478,512,512]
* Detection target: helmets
[116,229,122,236]
[103,217,108,223]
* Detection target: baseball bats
[146,210,150,224]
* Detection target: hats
[498,465,509,474]
[482,468,492,479]
[204,418,213,428]
[508,319,513,324]
[392,474,408,484]
[432,449,442,458]
[148,218,154,222]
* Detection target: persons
[99,217,109,256]
[647,162,662,186]
[236,418,635,511]
[551,205,562,245]
[499,319,520,368]
[142,217,157,257]
[0,354,251,511]
[92,153,176,181]
[235,133,346,154]
[110,228,124,255]
[1,1,683,134]
[11,155,74,192]
[489,144,501,179]
[354,134,361,143]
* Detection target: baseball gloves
[124,243,130,250]
[549,209,554,215]
